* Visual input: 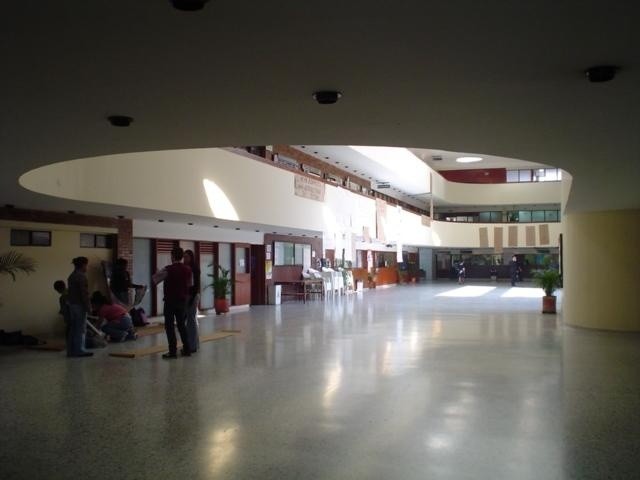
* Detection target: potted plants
[530,254,562,314]
[203,262,243,314]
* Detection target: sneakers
[160,343,199,360]
[64,345,95,360]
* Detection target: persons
[458,261,466,284]
[93,293,137,343]
[111,258,144,303]
[53,279,72,357]
[152,247,195,359]
[511,255,517,287]
[180,249,201,354]
[66,256,95,357]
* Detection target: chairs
[301,265,354,301]
